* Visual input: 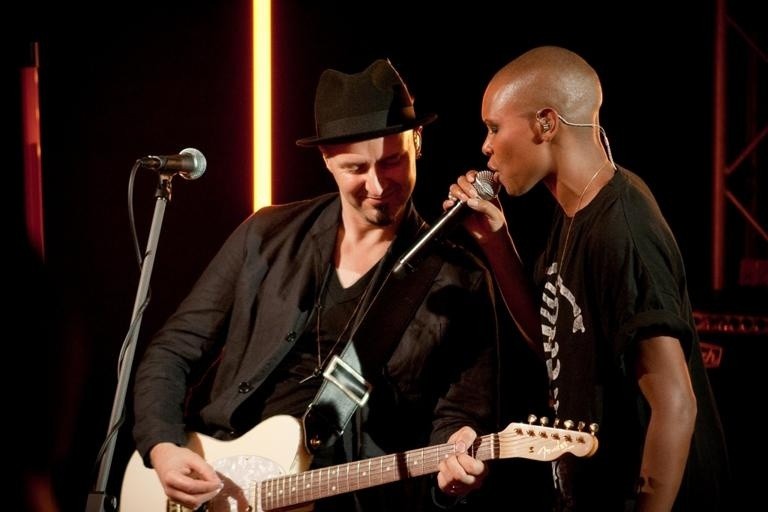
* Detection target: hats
[296,57,440,147]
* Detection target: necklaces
[547,159,610,344]
[298,279,371,384]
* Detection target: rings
[451,485,460,491]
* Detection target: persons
[133,60,498,510]
[442,46,728,511]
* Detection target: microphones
[391,170,501,273]
[139,148,207,182]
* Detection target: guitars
[116,413,598,512]
